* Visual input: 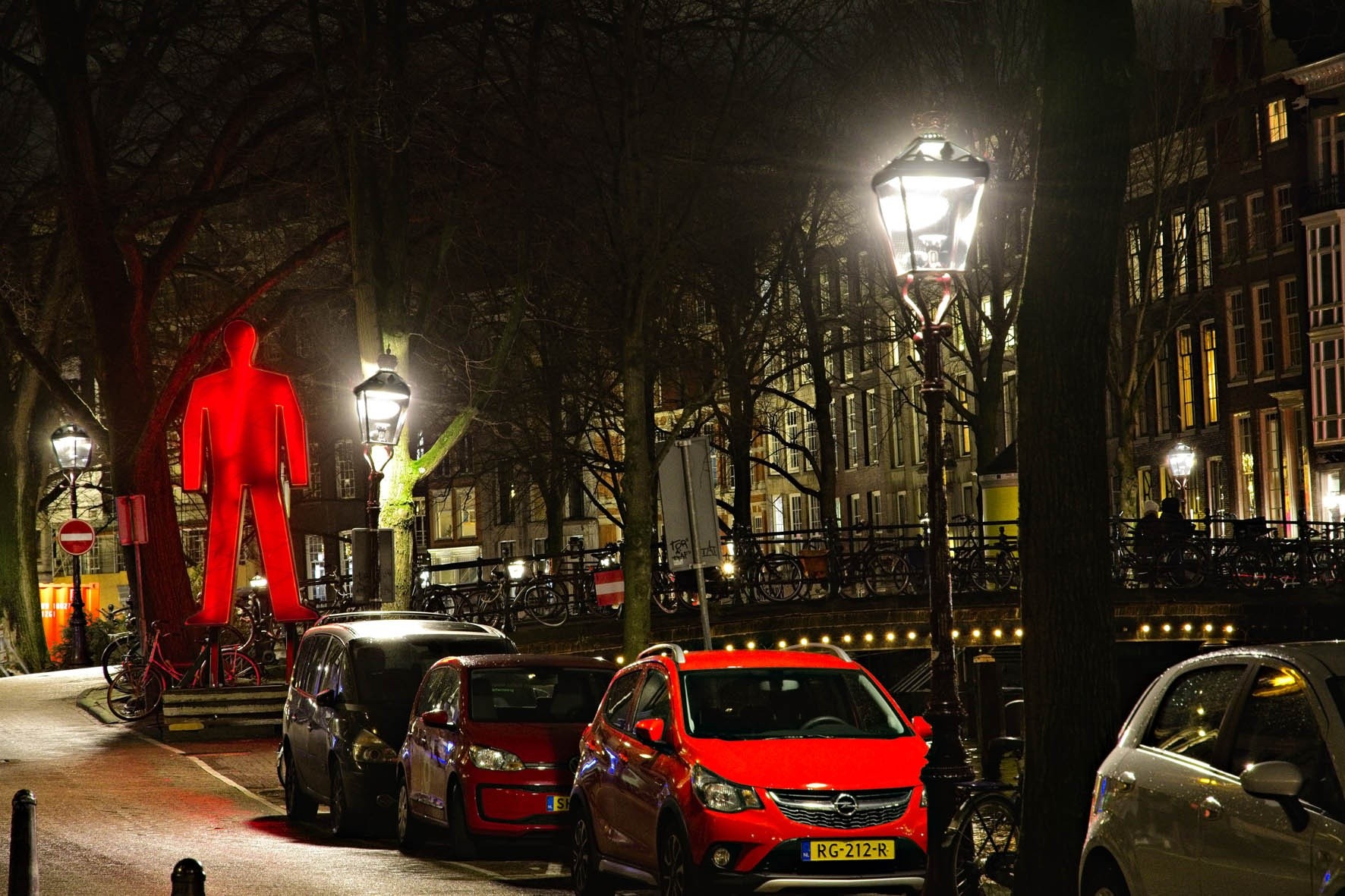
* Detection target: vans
[278,612,520,849]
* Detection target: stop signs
[58,519,96,556]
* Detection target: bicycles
[942,696,1025,896]
[102,598,305,722]
[719,517,1020,614]
[1105,511,1345,591]
[302,536,687,636]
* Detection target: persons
[1134,500,1160,586]
[1157,498,1192,560]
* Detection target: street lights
[868,82,992,896]
[49,406,95,668]
[1164,433,1196,524]
[354,341,411,619]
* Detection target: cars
[1076,640,1345,896]
[571,643,933,896]
[392,651,628,861]
